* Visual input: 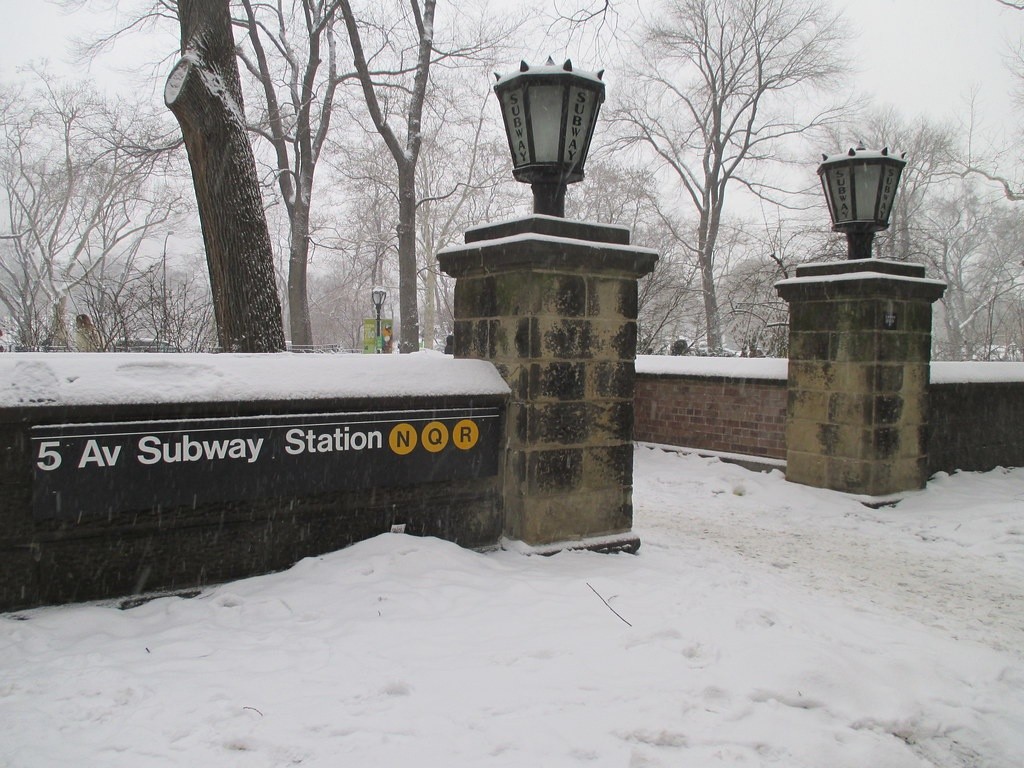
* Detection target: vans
[116,337,176,352]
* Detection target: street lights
[164,230,175,313]
[372,286,387,354]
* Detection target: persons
[75,314,101,352]
[445,335,453,354]
